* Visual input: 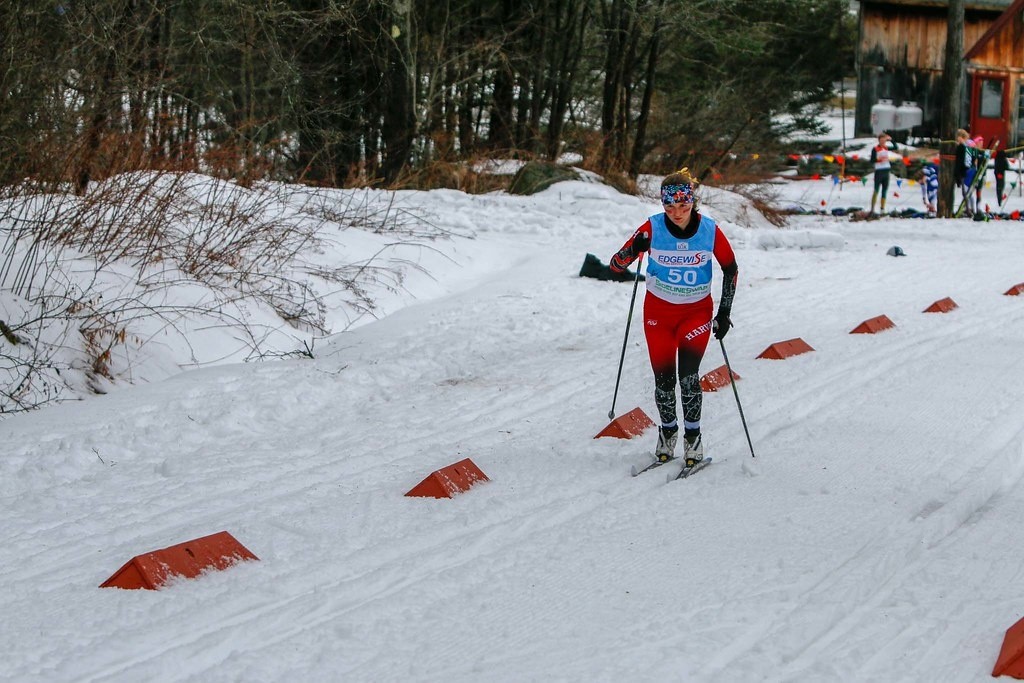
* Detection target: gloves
[631,232,650,256]
[713,309,733,340]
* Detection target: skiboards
[631,456,713,484]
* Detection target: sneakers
[683,433,703,463]
[656,426,679,462]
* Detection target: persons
[609,168,739,470]
[955,129,985,215]
[869,133,897,216]
[916,163,939,218]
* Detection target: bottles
[986,149,989,159]
